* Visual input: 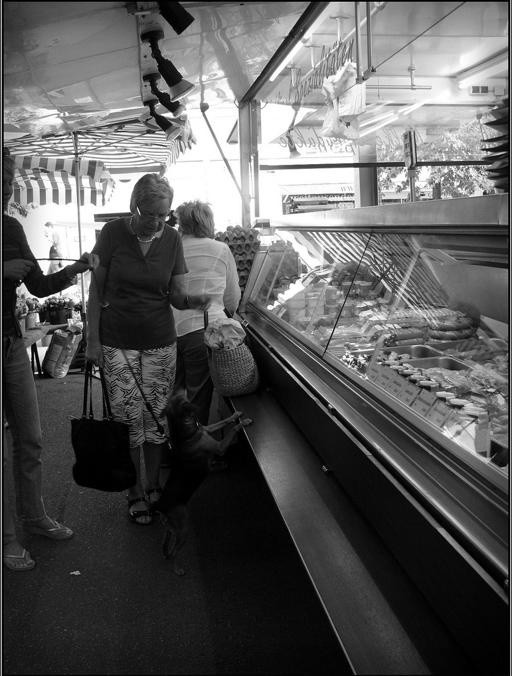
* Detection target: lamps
[127,1,195,144]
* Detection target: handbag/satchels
[71,418,137,492]
[205,339,259,398]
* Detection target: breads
[360,305,478,347]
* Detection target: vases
[17,312,77,336]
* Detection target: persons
[4,145,102,571]
[44,220,64,275]
[85,173,215,526]
[171,201,241,423]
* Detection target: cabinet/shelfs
[228,190,510,675]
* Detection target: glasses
[135,201,173,224]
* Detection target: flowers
[13,294,76,313]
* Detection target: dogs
[159,387,253,562]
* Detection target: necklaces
[127,212,157,244]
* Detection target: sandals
[23,515,74,540]
[143,485,163,516]
[126,493,153,525]
[2,548,37,571]
